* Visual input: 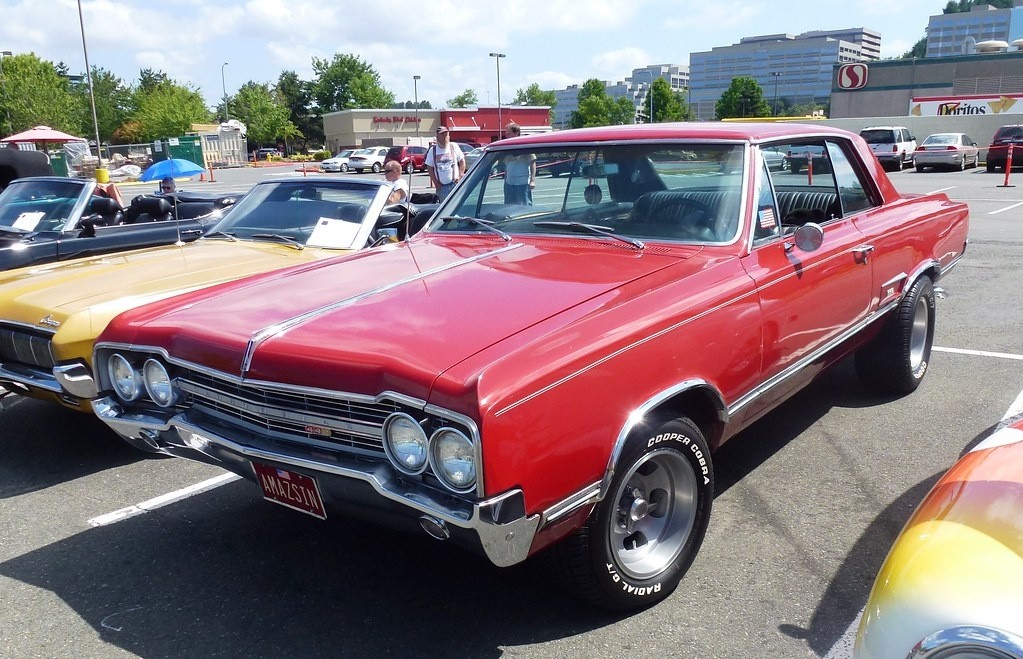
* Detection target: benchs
[630,192,848,231]
[173,202,214,219]
[403,203,552,222]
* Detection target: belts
[441,181,456,186]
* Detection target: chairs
[335,204,375,245]
[126,198,175,225]
[409,210,434,236]
[83,198,123,227]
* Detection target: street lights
[490,53,506,141]
[637,70,653,123]
[222,62,228,123]
[414,76,420,137]
[772,72,782,117]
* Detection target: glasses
[162,186,172,190]
[385,170,393,173]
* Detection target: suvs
[859,125,917,171]
[248,148,281,161]
[787,144,833,174]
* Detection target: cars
[986,125,1023,173]
[423,143,604,178]
[915,133,979,171]
[0,177,442,414]
[720,146,788,175]
[852,417,1022,659]
[320,149,364,172]
[348,147,391,173]
[384,146,428,174]
[93,122,970,608]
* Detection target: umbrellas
[0,125,87,153]
[139,154,207,182]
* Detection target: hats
[437,126,449,134]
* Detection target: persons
[385,161,408,206]
[425,126,466,201]
[502,123,537,205]
[156,177,176,195]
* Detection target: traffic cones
[199,172,207,182]
